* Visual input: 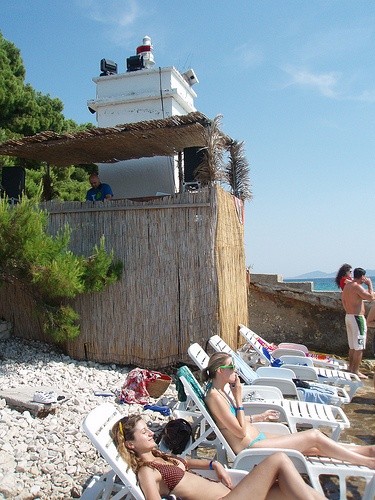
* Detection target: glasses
[91,182,98,185]
[215,362,235,372]
[347,271,352,273]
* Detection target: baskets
[145,373,171,398]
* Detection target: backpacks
[155,419,199,455]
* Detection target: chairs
[238,323,347,371]
[78,402,249,500]
[176,365,375,500]
[187,342,351,442]
[208,334,351,419]
[239,327,363,399]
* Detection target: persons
[86,174,114,201]
[342,268,375,378]
[335,264,354,290]
[111,414,330,500]
[201,352,375,469]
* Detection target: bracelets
[236,406,245,410]
[209,459,217,470]
[250,414,253,424]
